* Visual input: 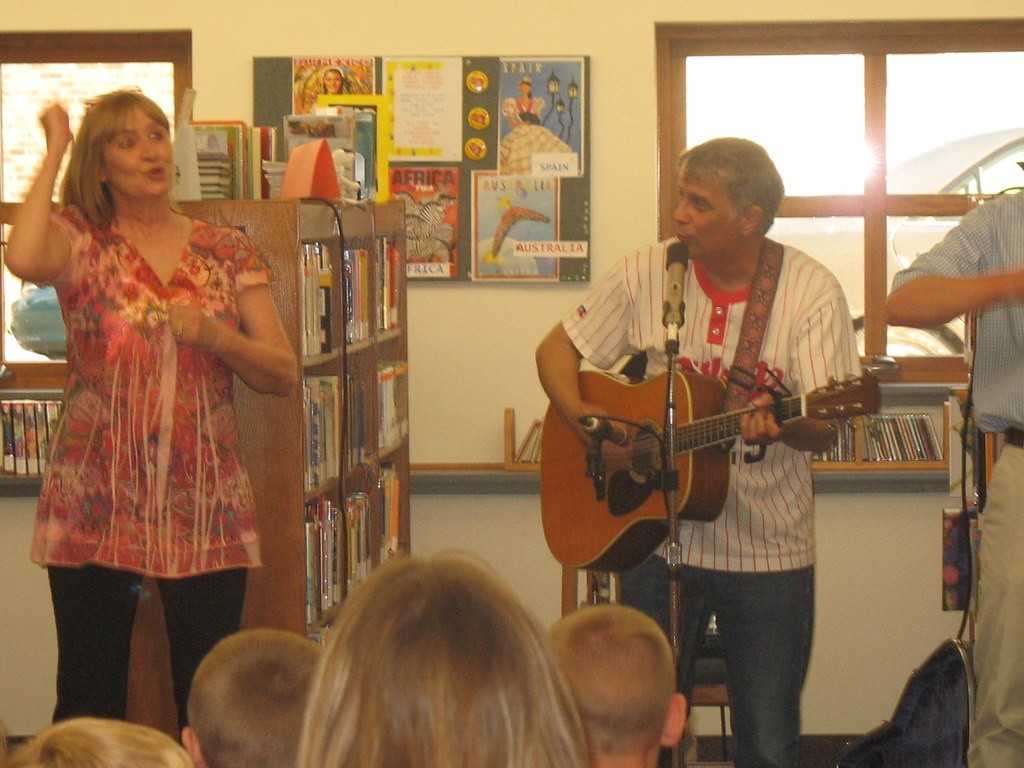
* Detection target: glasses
[84,85,144,105]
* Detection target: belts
[1004,426,1024,448]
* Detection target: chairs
[556,566,733,768]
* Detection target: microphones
[580,414,631,449]
[663,243,689,356]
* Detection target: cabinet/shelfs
[119,197,409,744]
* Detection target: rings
[176,321,183,336]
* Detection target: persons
[296,556,590,768]
[4,92,298,749]
[182,628,320,768]
[547,605,686,768]
[885,189,1024,768]
[12,717,194,768]
[536,135,863,768]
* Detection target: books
[175,95,390,201]
[810,414,943,461]
[301,238,400,357]
[305,462,400,646]
[303,361,407,492]
[0,400,63,475]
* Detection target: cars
[764,123,1024,360]
[7,277,67,361]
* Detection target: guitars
[537,365,885,577]
